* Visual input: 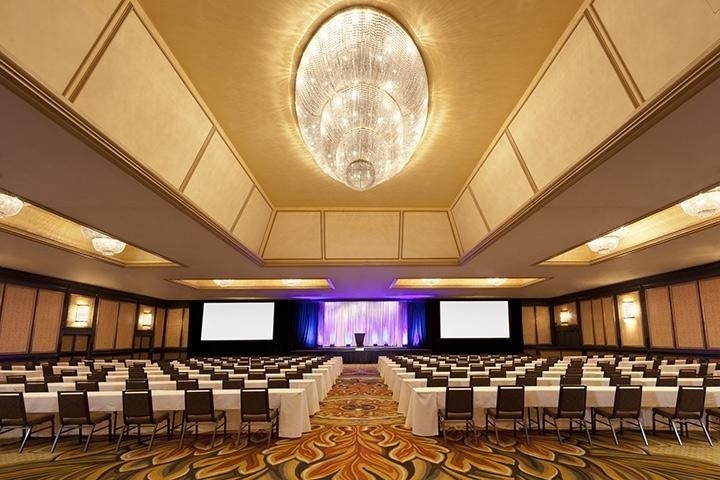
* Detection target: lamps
[76,304,90,322]
[286,0,431,192]
[621,301,635,320]
[79,225,129,258]
[680,189,720,220]
[142,312,152,326]
[585,227,629,254]
[559,309,569,326]
[0,192,29,220]
